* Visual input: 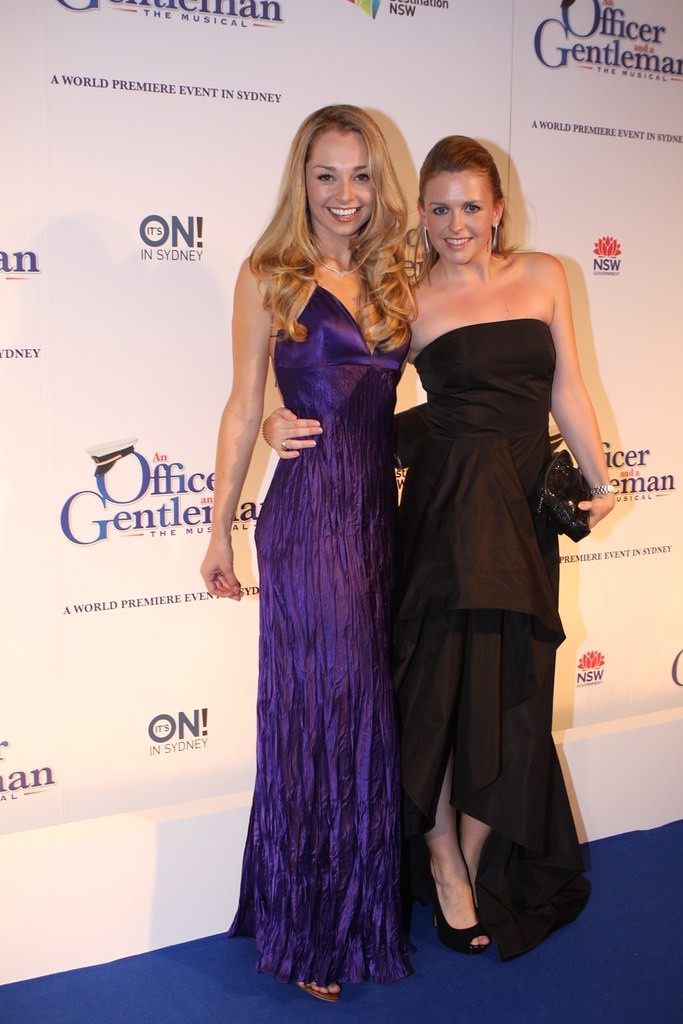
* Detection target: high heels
[428,860,488,955]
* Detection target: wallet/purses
[533,450,590,543]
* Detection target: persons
[200,103,419,1003]
[263,134,616,960]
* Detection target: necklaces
[320,262,347,278]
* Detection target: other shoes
[297,982,339,1002]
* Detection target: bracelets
[262,417,273,448]
[590,485,614,496]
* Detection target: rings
[281,442,286,450]
